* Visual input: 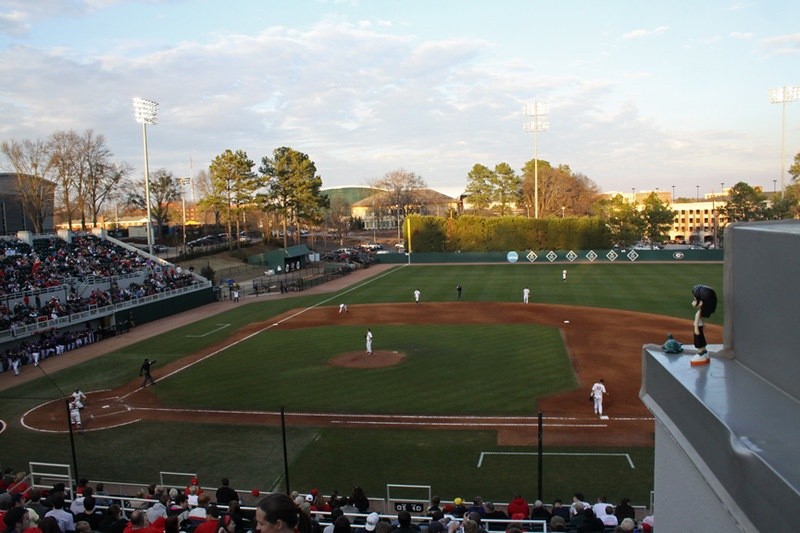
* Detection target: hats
[309,488,318,495]
[192,478,199,485]
[252,489,260,496]
[0,493,11,503]
[198,493,210,504]
[365,512,379,531]
[575,492,585,501]
[305,494,313,502]
[454,497,462,505]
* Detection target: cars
[154,244,168,253]
[273,226,310,239]
[335,241,382,256]
[185,230,262,248]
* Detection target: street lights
[773,179,777,193]
[721,183,725,201]
[525,204,532,217]
[696,185,700,203]
[632,187,635,202]
[767,85,800,199]
[524,98,557,216]
[133,96,161,242]
[561,206,565,218]
[672,185,676,203]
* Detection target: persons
[523,287,530,304]
[413,289,421,303]
[339,304,348,313]
[690,285,717,365]
[591,379,609,417]
[68,396,82,430]
[71,388,86,410]
[563,268,567,282]
[365,329,373,355]
[230,244,382,303]
[456,283,462,298]
[0,234,205,377]
[0,468,654,533]
[140,359,157,386]
